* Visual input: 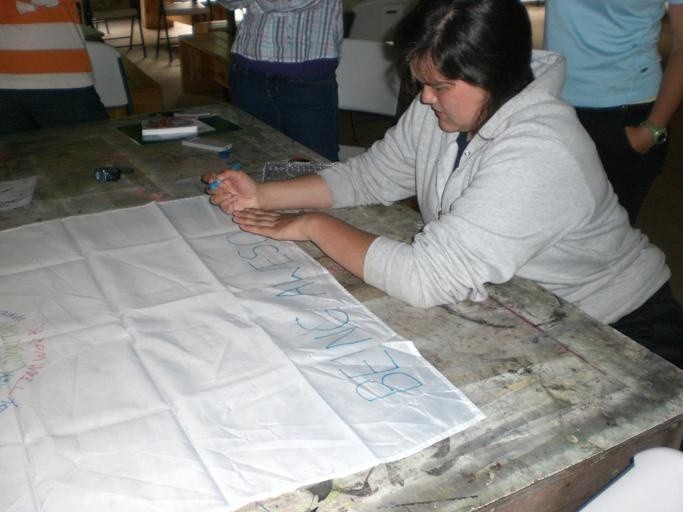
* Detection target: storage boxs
[179,31,232,100]
[106,53,165,118]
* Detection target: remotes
[182,136,232,152]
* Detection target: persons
[538,0,683,230]
[211,0,345,163]
[0,1,112,141]
[197,1,683,375]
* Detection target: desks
[0,101,683,511]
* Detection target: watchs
[639,118,668,147]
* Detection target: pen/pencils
[163,111,211,118]
[207,164,242,191]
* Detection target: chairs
[88,2,148,57]
[155,0,215,62]
